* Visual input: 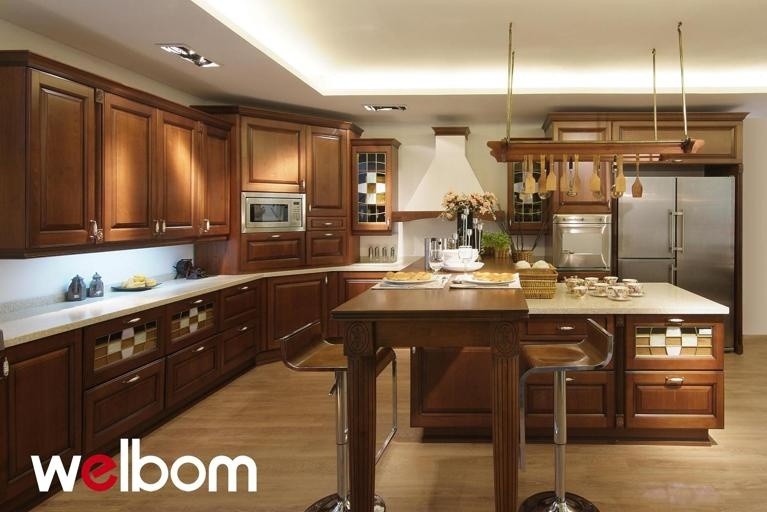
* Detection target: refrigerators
[617,176,736,352]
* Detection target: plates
[464,274,516,284]
[382,276,433,284]
[112,280,168,291]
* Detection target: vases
[457,205,481,250]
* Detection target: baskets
[517,259,559,300]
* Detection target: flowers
[435,190,500,226]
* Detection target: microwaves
[241,192,306,233]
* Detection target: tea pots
[68,273,104,301]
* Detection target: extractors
[401,128,492,214]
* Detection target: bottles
[369,247,395,259]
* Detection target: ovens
[552,214,611,272]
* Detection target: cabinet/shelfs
[187,104,401,274]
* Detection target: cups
[424,237,485,273]
[565,276,644,301]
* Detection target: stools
[518,317,615,512]
[278,317,397,512]
